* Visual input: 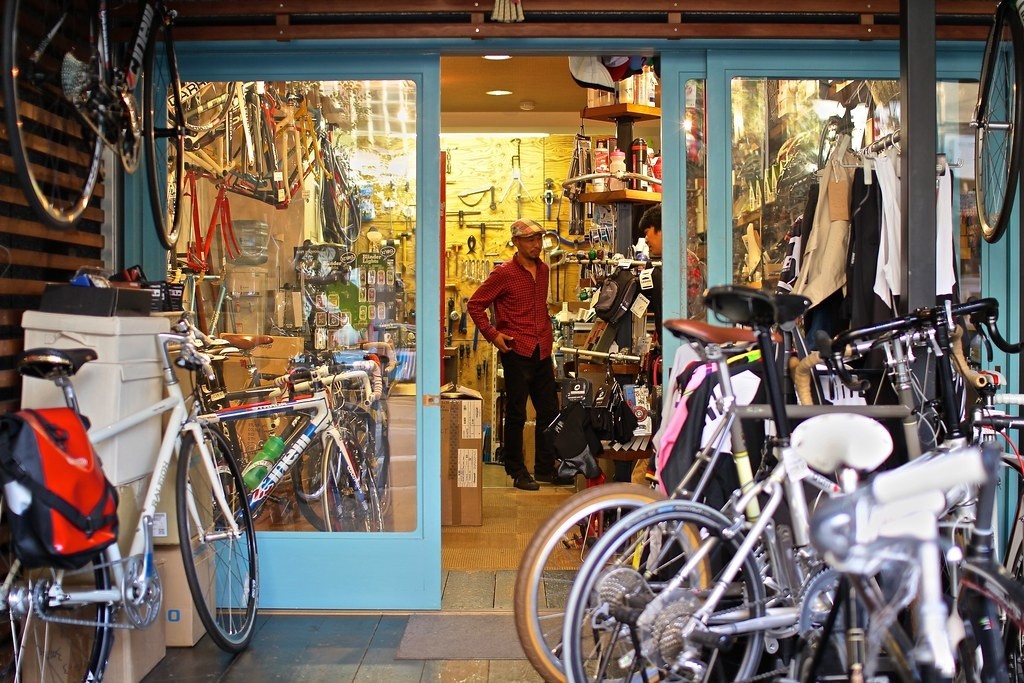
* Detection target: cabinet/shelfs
[169,78,323,530]
[551,79,867,482]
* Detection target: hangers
[776,126,964,194]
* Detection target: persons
[467,219,575,490]
[635,205,662,346]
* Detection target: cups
[553,347,564,382]
[630,138,650,191]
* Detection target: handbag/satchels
[591,361,639,445]
[594,267,638,323]
[2,408,121,568]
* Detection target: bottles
[226,220,270,265]
[609,150,626,190]
[240,435,287,489]
[633,65,656,107]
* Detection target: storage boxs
[21,281,307,682]
[375,380,562,533]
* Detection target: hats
[510,218,547,237]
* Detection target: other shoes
[534,472,557,482]
[513,469,541,490]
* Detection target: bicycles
[185,318,402,532]
[969,0,1024,244]
[0,316,261,682]
[166,82,364,265]
[514,284,1024,683]
[1,1,199,250]
